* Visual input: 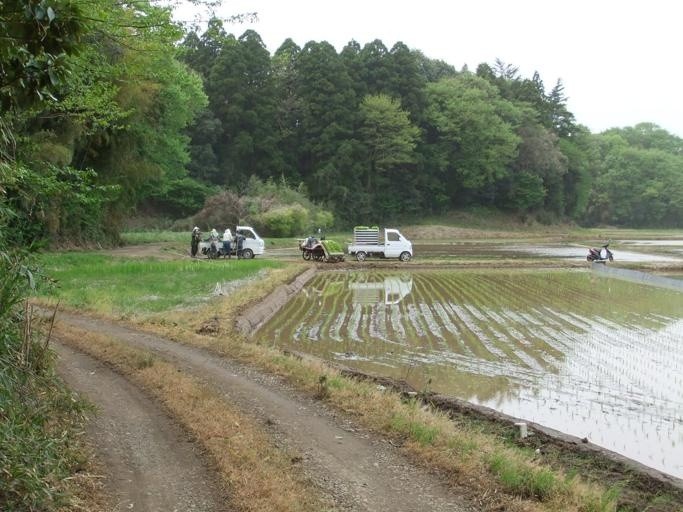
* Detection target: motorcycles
[586,244,615,262]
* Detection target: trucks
[346,228,414,263]
[196,225,265,260]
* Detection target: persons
[191,225,246,260]
